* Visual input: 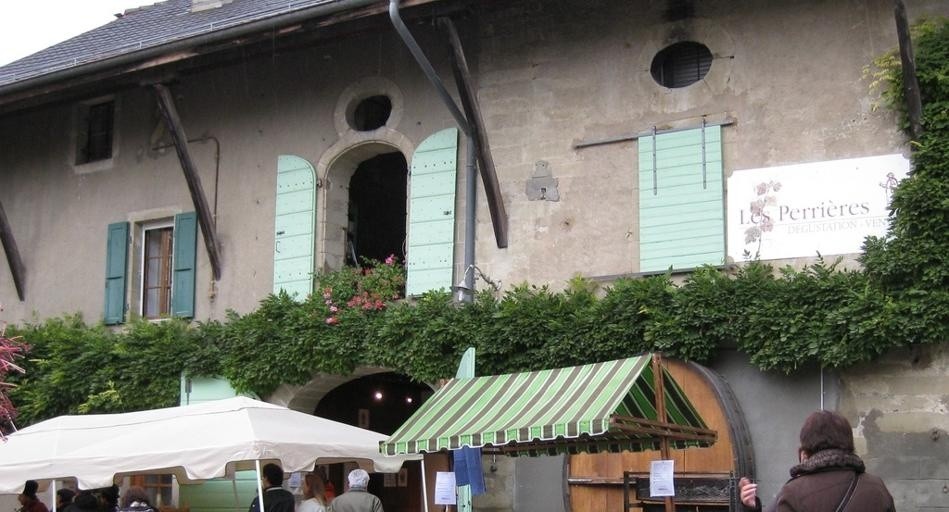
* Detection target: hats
[22,480,38,495]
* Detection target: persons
[54,488,81,511]
[248,462,295,511]
[13,479,51,511]
[93,483,121,511]
[296,473,331,512]
[736,443,810,512]
[118,484,160,512]
[775,409,898,512]
[73,493,99,511]
[328,468,385,512]
